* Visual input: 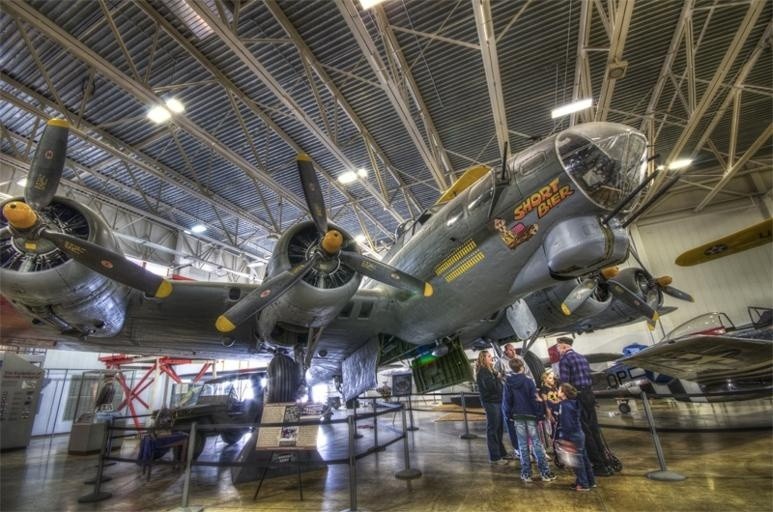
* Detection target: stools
[140,432,188,481]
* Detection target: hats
[557,337,573,346]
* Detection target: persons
[553,336,617,477]
[537,367,559,457]
[474,349,511,465]
[499,357,558,484]
[493,342,538,457]
[546,380,597,491]
[93,369,120,414]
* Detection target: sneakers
[569,483,590,492]
[592,463,610,476]
[542,472,557,482]
[501,453,512,459]
[513,446,555,463]
[520,472,532,482]
[490,459,508,466]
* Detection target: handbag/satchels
[553,439,581,468]
[610,457,622,472]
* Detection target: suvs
[141,369,302,465]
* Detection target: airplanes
[511,306,772,414]
[0,119,695,424]
[339,347,625,406]
